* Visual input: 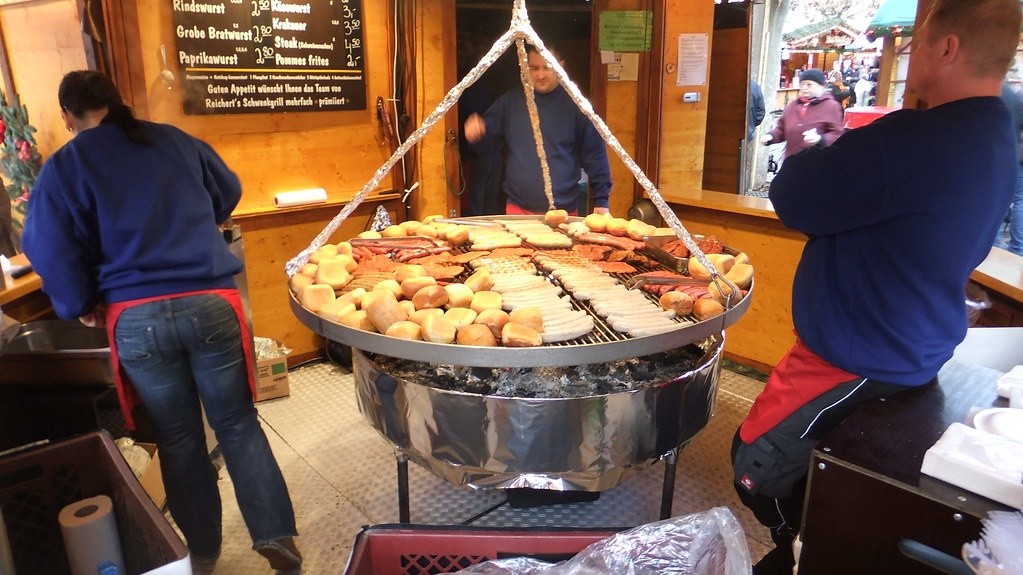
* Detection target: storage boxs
[256,356,291,401]
[920,422,1023,511]
[342,528,727,575]
[0,429,194,575]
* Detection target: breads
[546,210,754,321]
[289,214,544,347]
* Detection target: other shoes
[255,536,302,570]
[189,547,220,560]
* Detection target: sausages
[340,218,746,343]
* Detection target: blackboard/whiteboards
[172,0,367,115]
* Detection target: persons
[462,38,610,219]
[746,78,766,147]
[759,69,844,161]
[20,70,303,575]
[823,58,881,107]
[729,1,1023,575]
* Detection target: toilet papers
[57,494,126,575]
[273,188,328,208]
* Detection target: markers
[378,188,398,195]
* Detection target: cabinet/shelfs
[797,326,1023,575]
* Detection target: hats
[800,68,825,86]
